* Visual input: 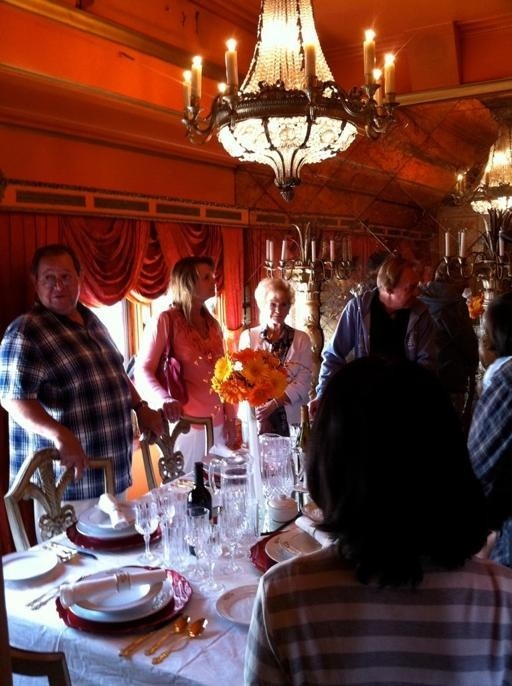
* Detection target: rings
[168,405,173,409]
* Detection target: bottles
[187,462,213,556]
[296,405,312,454]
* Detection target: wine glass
[134,500,159,564]
[161,434,308,591]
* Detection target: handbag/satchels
[125,353,189,406]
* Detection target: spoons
[43,543,80,564]
[144,612,208,664]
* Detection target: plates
[56,566,194,639]
[265,530,320,564]
[3,551,58,581]
[65,500,162,552]
[215,584,259,627]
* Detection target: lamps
[452,117,512,218]
[182,1,398,199]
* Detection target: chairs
[4,409,214,552]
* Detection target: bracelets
[270,396,281,408]
[135,399,148,413]
[226,417,236,423]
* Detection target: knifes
[50,541,99,560]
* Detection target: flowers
[209,348,288,406]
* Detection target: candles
[444,228,467,259]
[264,237,349,268]
[499,231,504,256]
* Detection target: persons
[302,258,440,426]
[137,255,237,475]
[244,355,511,683]
[415,255,481,420]
[1,240,166,550]
[461,360,512,573]
[231,275,318,433]
[479,290,511,388]
